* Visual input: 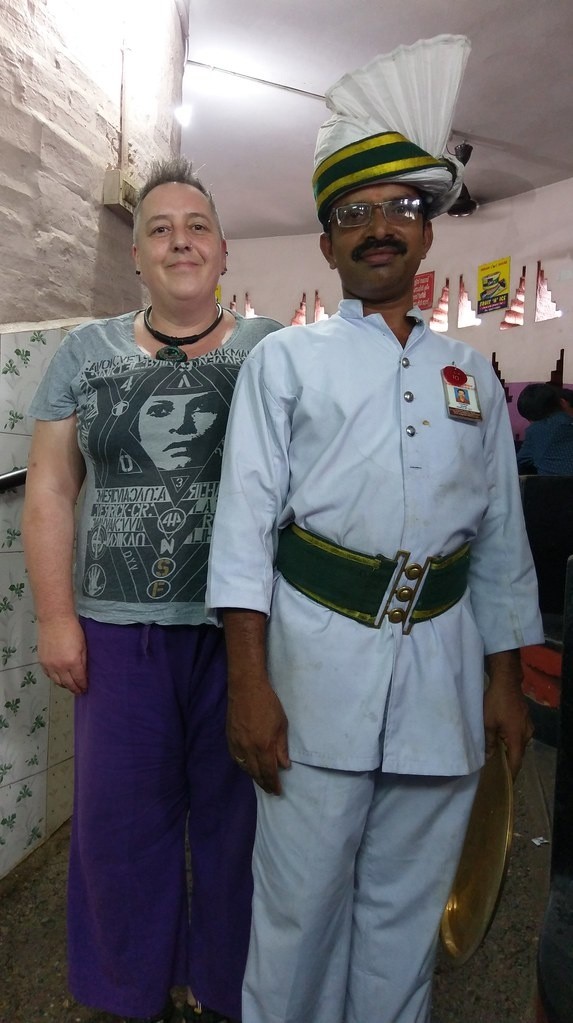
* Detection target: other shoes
[116,994,179,1023]
[182,990,240,1023]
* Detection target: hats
[309,30,476,233]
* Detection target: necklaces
[144,301,223,362]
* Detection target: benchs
[518,475,573,752]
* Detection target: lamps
[446,141,477,218]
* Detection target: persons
[204,33,545,1023]
[22,159,283,1023]
[516,383,573,476]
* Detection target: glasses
[326,195,428,235]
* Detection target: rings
[235,756,245,763]
